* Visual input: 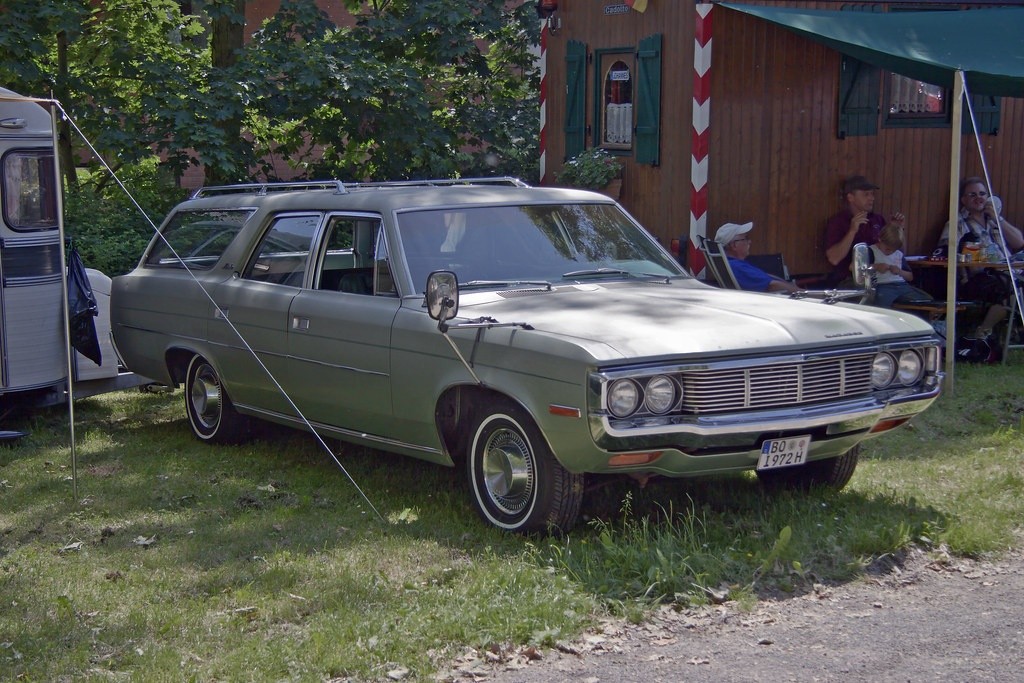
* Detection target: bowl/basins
[959,254,972,262]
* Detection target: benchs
[889,304,967,323]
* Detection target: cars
[108,176,947,540]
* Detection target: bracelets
[994,216,1004,224]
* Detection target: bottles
[979,231,990,261]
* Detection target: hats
[714,222,753,246]
[845,176,880,193]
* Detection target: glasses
[964,193,989,199]
[733,236,750,243]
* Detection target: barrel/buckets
[66,268,119,380]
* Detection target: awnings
[713,0,1024,395]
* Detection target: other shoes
[974,326,992,338]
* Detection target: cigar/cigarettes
[861,210,864,214]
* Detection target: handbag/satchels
[955,334,1003,364]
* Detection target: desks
[908,260,1024,365]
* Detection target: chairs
[698,234,740,293]
[744,253,789,281]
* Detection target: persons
[715,175,1024,361]
